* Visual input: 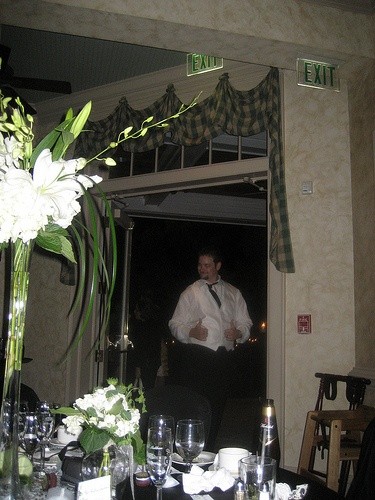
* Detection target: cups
[239,455,277,500]
[48,403,61,434]
[217,448,252,480]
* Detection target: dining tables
[0,441,339,500]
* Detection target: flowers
[47,376,146,477]
[0,91,204,371]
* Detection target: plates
[171,451,216,467]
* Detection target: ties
[206,282,222,308]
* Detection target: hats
[0,336,33,364]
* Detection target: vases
[81,444,129,488]
[0,233,36,500]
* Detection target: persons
[168,246,253,453]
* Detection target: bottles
[255,399,280,474]
[148,414,175,478]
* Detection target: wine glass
[32,400,55,474]
[146,427,173,500]
[174,419,205,474]
[15,411,40,472]
[0,398,29,450]
